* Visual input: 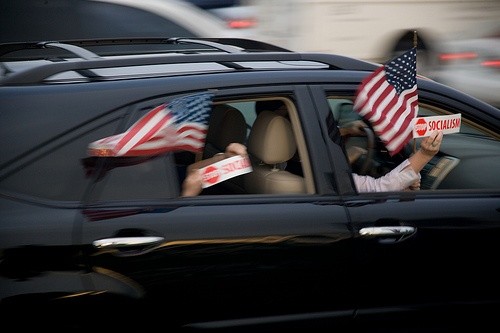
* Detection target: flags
[88,90,214,158]
[352,46,420,158]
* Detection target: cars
[1,0,229,45]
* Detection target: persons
[178,140,248,197]
[338,120,370,166]
[351,130,444,193]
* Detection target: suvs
[0,34,497,333]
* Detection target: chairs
[223,110,307,194]
